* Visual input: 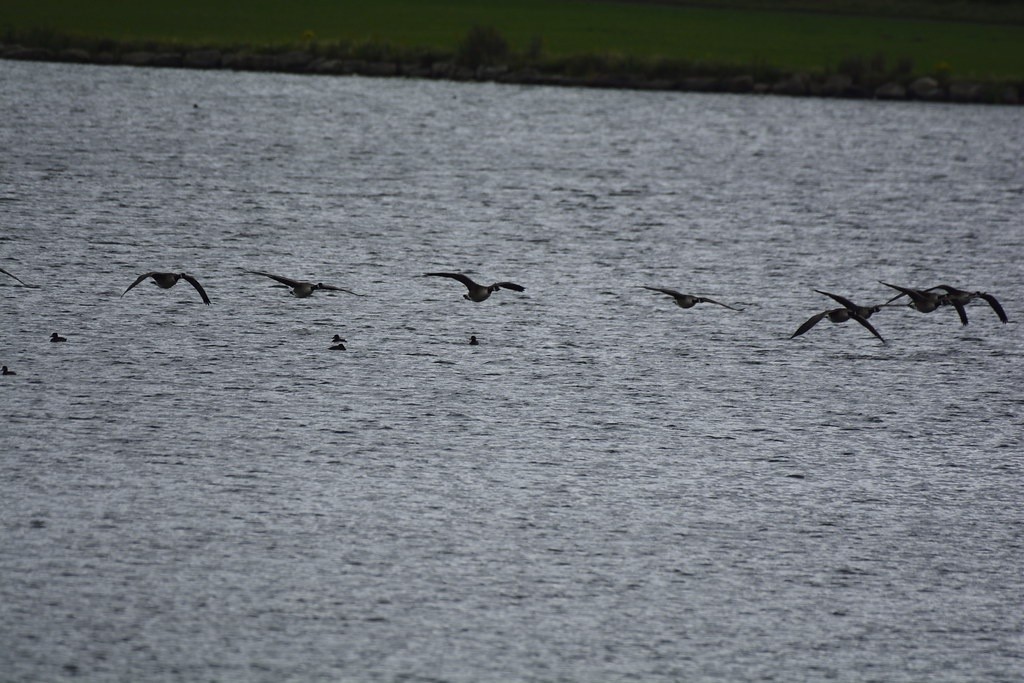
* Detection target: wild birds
[641,285,744,312]
[788,280,1009,343]
[48,332,69,342]
[327,334,347,350]
[468,336,479,345]
[120,271,212,306]
[422,271,526,303]
[0,365,17,375]
[243,271,367,298]
[0,266,26,286]
